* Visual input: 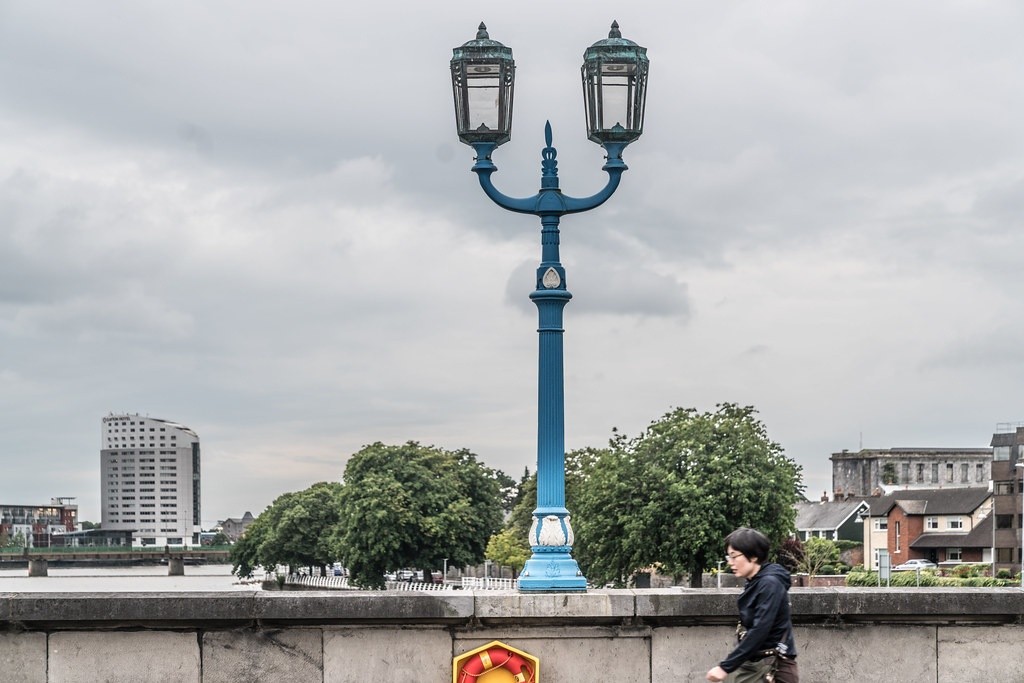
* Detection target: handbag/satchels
[722,649,780,683]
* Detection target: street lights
[449,15,650,592]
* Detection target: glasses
[725,552,743,563]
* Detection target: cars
[894,558,938,573]
[383,567,443,583]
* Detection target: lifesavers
[455,645,537,683]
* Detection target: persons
[397,571,417,582]
[288,570,306,580]
[706,526,799,683]
